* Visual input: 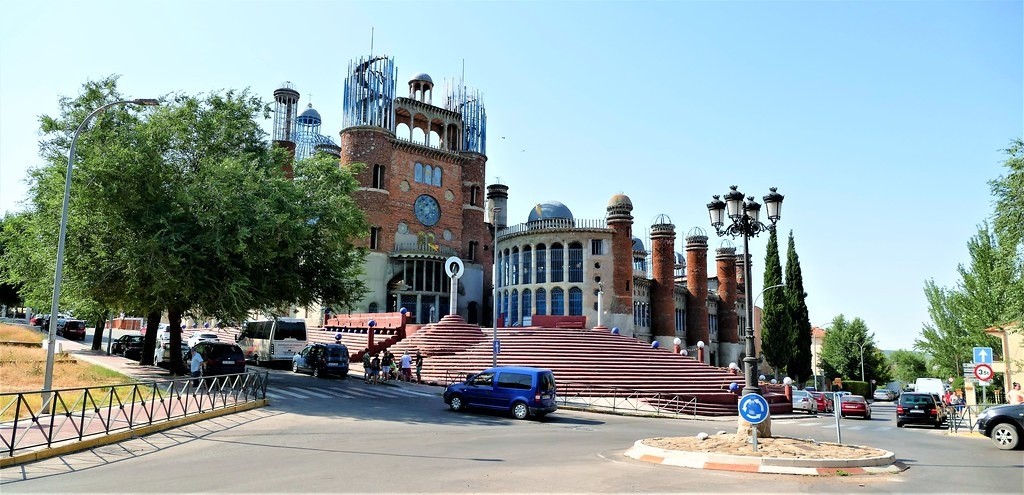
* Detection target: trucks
[914,378,946,400]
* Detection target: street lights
[491,206,502,369]
[752,283,789,338]
[812,320,846,392]
[705,183,787,438]
[846,338,870,381]
[41,97,160,419]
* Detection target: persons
[372,353,380,384]
[381,351,397,383]
[416,349,422,384]
[1007,382,1024,404]
[945,391,965,418]
[140,326,146,334]
[187,349,203,388]
[396,350,413,380]
[30,318,36,325]
[363,349,371,383]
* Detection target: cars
[62,320,86,340]
[139,323,185,340]
[791,390,819,415]
[809,391,852,413]
[839,395,873,420]
[874,390,901,401]
[927,391,948,422]
[41,314,68,336]
[110,334,145,362]
[29,314,45,328]
[187,332,220,349]
[154,340,190,367]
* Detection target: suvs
[976,402,1024,451]
[897,391,941,429]
[183,341,245,382]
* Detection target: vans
[444,367,559,420]
[291,343,349,378]
[887,383,905,399]
[234,317,308,366]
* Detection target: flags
[429,243,439,251]
[536,205,541,215]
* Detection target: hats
[191,348,196,352]
